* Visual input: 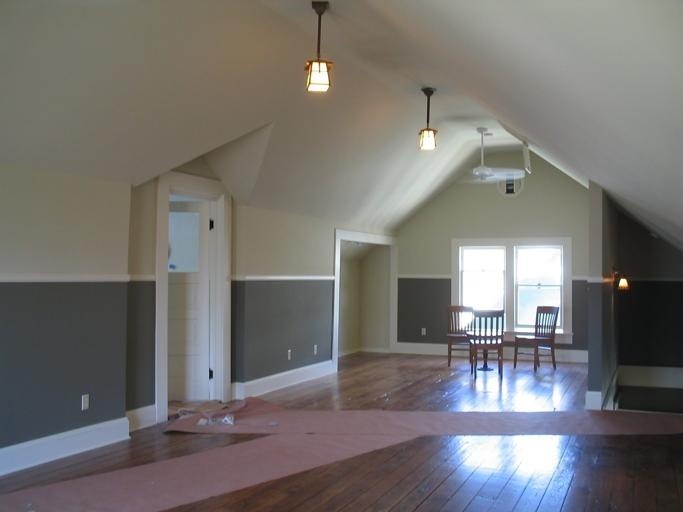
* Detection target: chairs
[514,306,561,373]
[466,310,507,377]
[445,306,478,367]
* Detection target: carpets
[0,433,415,512]
[160,394,683,439]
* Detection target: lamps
[303,1,331,93]
[415,86,440,151]
[611,268,630,289]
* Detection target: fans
[446,128,525,186]
